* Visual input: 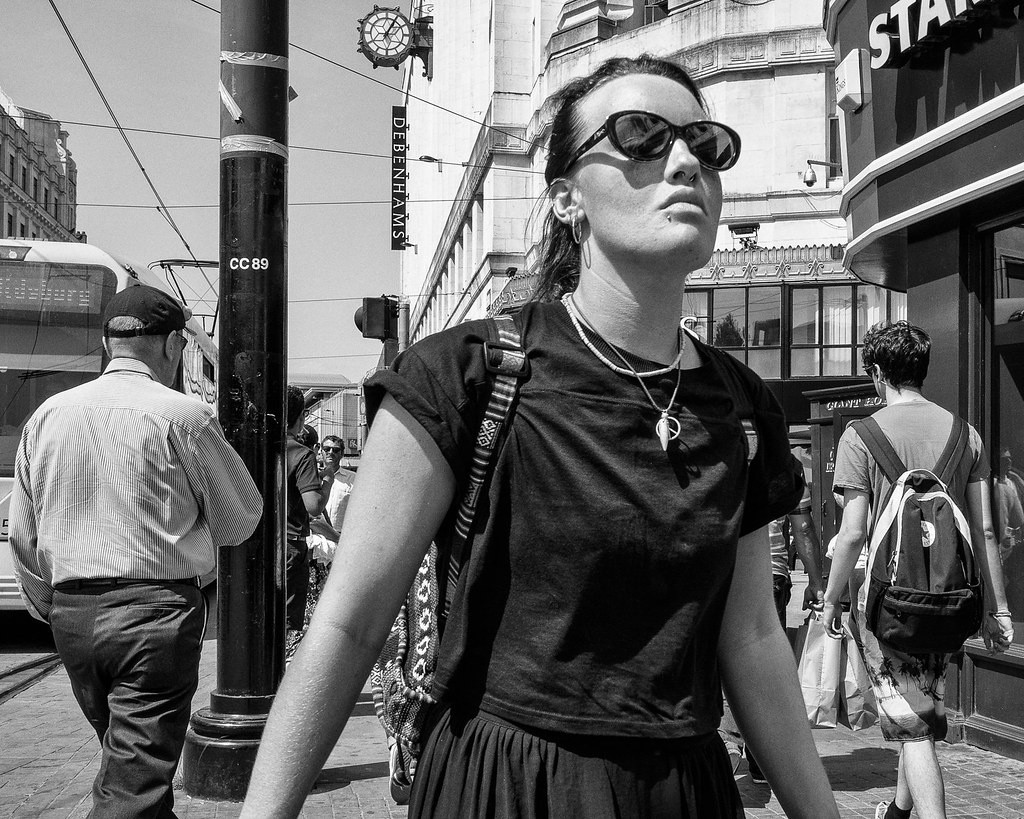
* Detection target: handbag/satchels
[787,608,880,732]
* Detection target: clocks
[356,4,434,81]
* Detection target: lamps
[419,155,442,172]
[729,222,760,247]
[505,267,517,277]
[401,243,418,254]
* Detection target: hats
[102,284,192,337]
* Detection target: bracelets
[990,612,1012,617]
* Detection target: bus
[0,238,220,611]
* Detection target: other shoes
[744,744,768,784]
[726,744,743,776]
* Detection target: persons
[286,386,355,633]
[237,54,845,819]
[732,320,1014,819]
[7,284,264,819]
[993,446,1024,596]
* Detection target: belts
[288,535,306,541]
[56,576,201,588]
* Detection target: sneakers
[875,800,892,819]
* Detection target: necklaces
[557,288,689,450]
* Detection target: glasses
[563,109,741,171]
[316,443,320,450]
[176,331,189,351]
[303,409,310,417]
[322,446,343,454]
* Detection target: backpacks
[848,411,983,656]
[370,314,758,805]
